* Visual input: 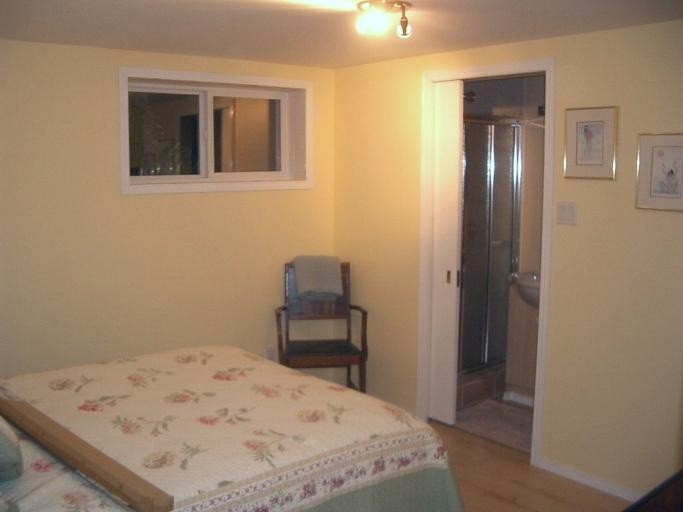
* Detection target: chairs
[274,261,368,394]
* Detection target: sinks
[515,271,540,305]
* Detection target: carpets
[455,394,533,454]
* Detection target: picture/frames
[561,105,683,213]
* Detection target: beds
[1,345,459,511]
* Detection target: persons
[581,124,595,160]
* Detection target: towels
[292,255,344,299]
